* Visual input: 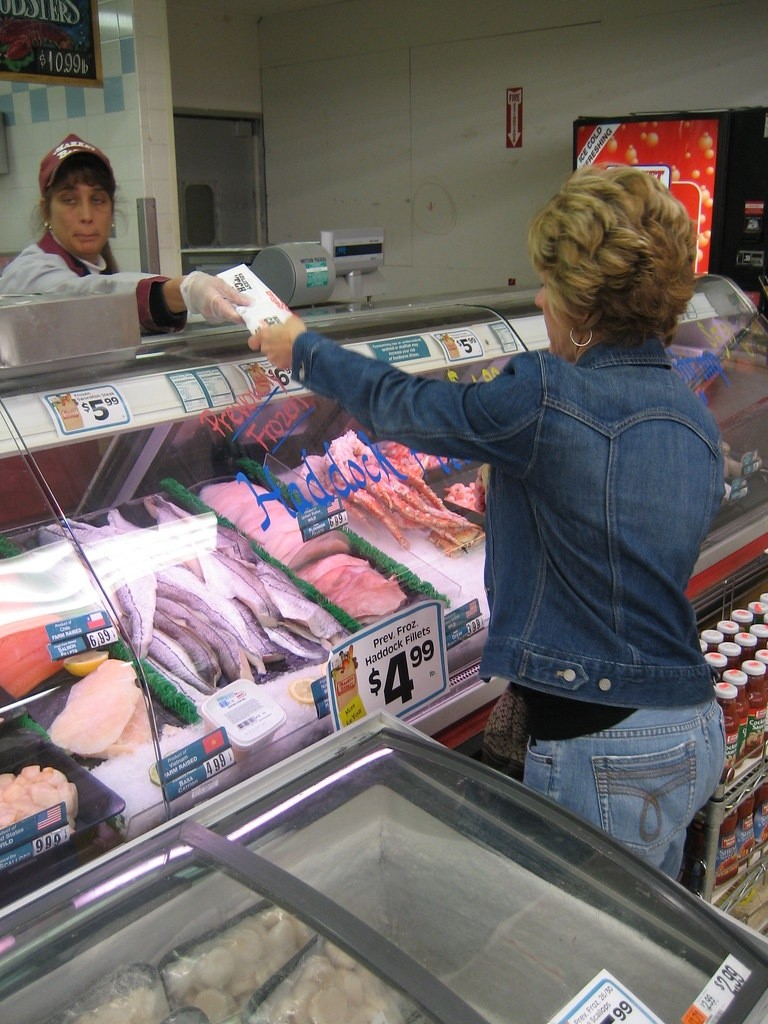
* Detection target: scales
[179,226,387,303]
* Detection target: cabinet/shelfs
[676,740,768,936]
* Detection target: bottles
[697,591,768,881]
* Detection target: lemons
[63,651,110,676]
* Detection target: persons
[0,134,251,336]
[245,163,727,884]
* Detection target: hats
[37,134,119,198]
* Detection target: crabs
[301,430,486,559]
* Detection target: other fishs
[37,494,348,697]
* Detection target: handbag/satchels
[482,686,533,781]
